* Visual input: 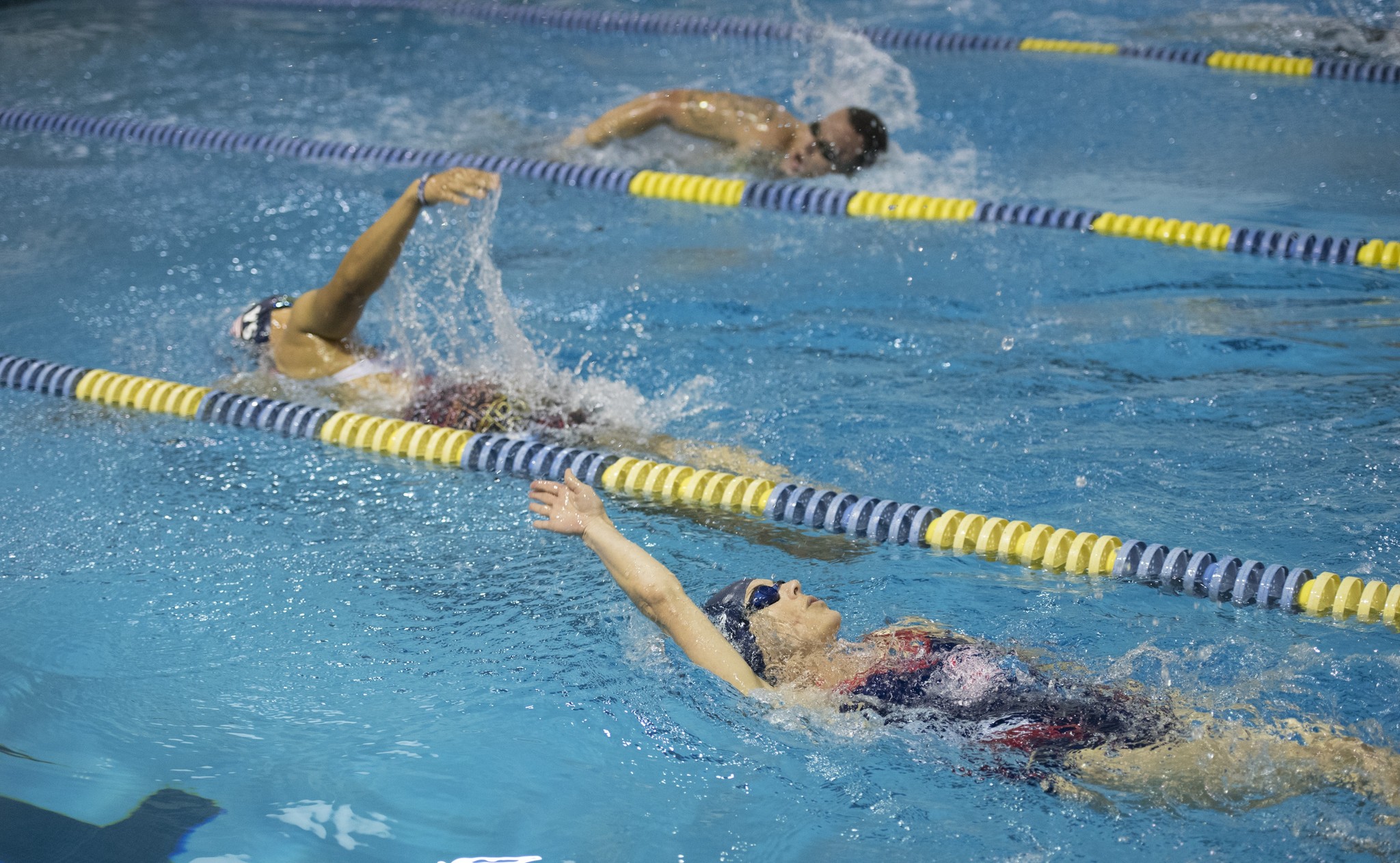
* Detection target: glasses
[745,580,785,616]
[272,301,292,308]
[810,122,834,161]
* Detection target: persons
[525,468,1400,822]
[216,164,795,483]
[561,89,888,180]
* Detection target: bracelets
[417,170,436,205]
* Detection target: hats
[701,578,766,674]
[230,294,294,343]
